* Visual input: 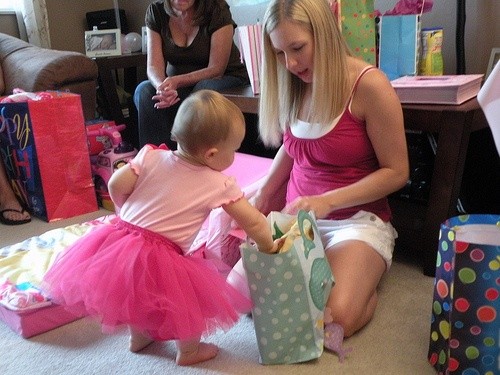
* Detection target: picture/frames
[85,28,121,57]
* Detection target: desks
[91,50,149,137]
[385,98,500,277]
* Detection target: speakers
[86,9,128,33]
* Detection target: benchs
[218,84,260,112]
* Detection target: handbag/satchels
[0,88,99,223]
[428,214,500,375]
[238,209,336,366]
[336,0,425,81]
[237,23,262,94]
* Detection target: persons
[231,0,410,338]
[41,89,282,366]
[133,0,248,151]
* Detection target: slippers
[0,207,31,225]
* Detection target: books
[390,72,484,106]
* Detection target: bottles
[420,27,443,75]
